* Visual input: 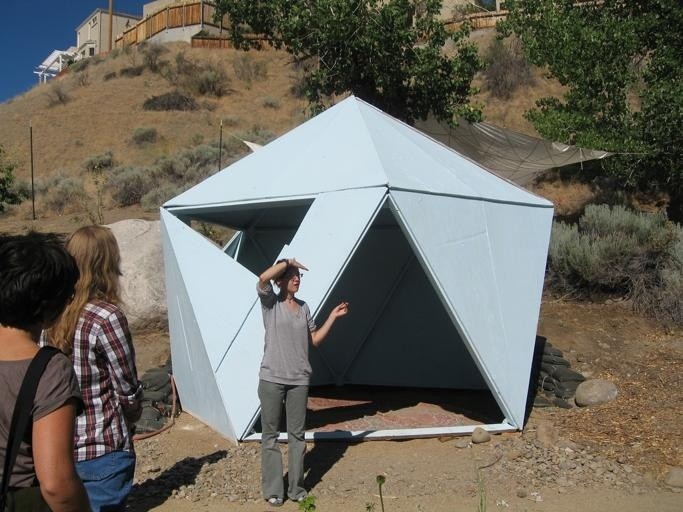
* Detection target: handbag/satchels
[3,486,51,511]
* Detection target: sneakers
[269,498,283,507]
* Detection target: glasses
[285,273,304,279]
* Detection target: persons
[256,258,350,507]
[39,224,144,512]
[0,228,94,512]
